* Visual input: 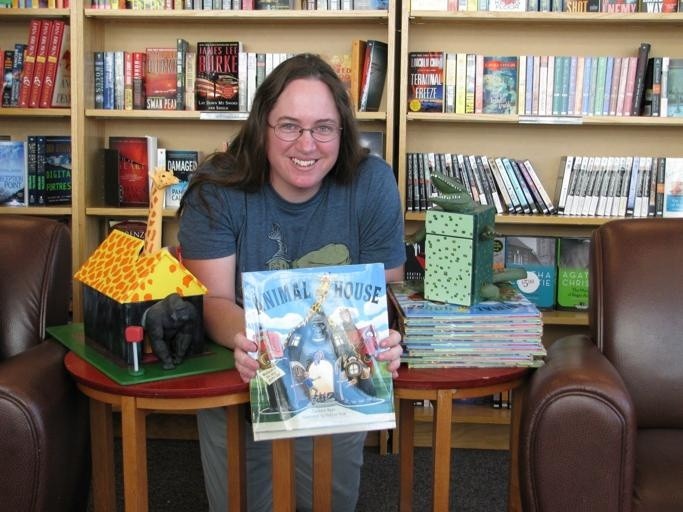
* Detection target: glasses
[266,122,342,143]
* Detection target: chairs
[1,213,76,511]
[518,210,681,509]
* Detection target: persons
[173,51,407,511]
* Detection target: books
[495,233,592,313]
[233,260,398,444]
[388,271,547,374]
[2,20,71,108]
[96,136,201,206]
[1,1,68,9]
[411,1,680,12]
[410,154,682,219]
[410,44,682,117]
[92,0,389,11]
[2,135,72,206]
[95,39,387,112]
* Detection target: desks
[310,361,530,512]
[59,350,295,510]
